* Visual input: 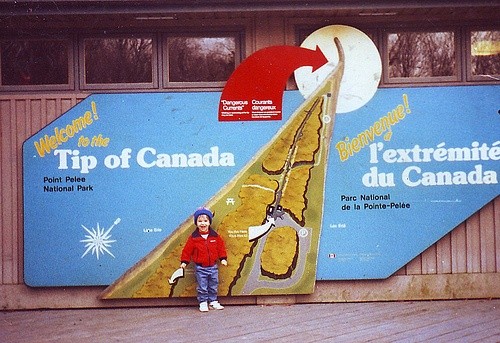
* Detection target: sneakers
[199,302,209,311]
[209,301,224,310]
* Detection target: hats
[194,209,212,225]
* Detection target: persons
[180,207,228,312]
